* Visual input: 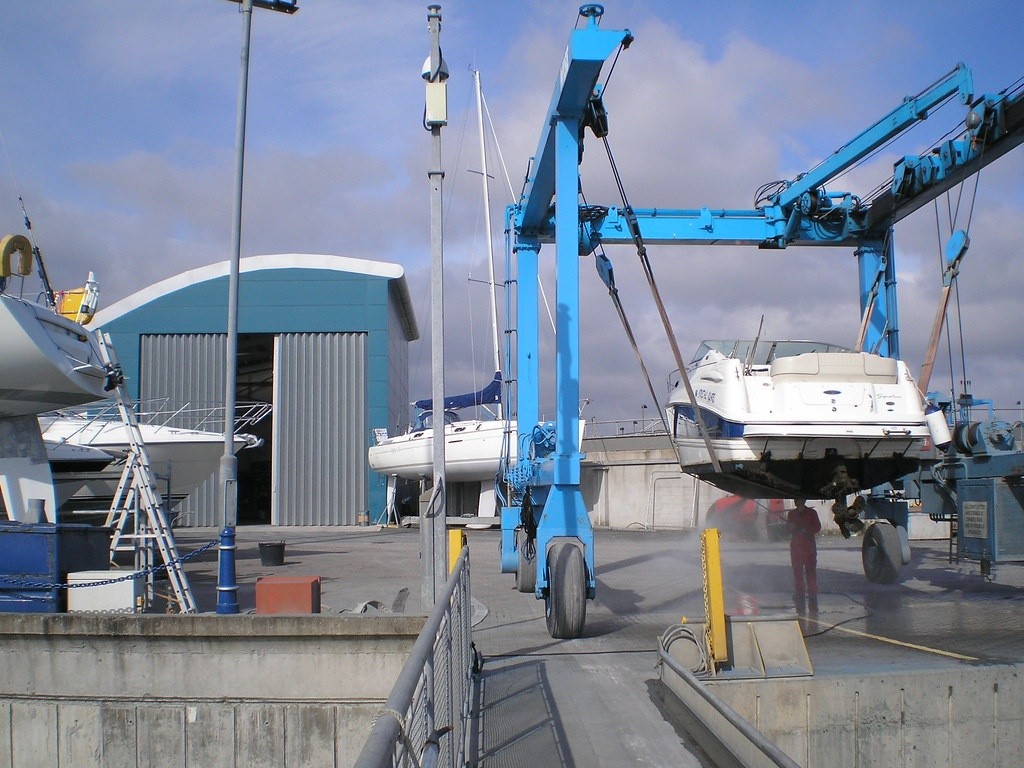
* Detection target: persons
[787,491,821,615]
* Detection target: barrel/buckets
[259,540,286,567]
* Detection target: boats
[35,396,275,520]
[664,338,953,502]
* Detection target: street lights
[633,421,638,433]
[620,427,624,435]
[641,404,647,434]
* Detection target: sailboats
[366,77,586,487]
